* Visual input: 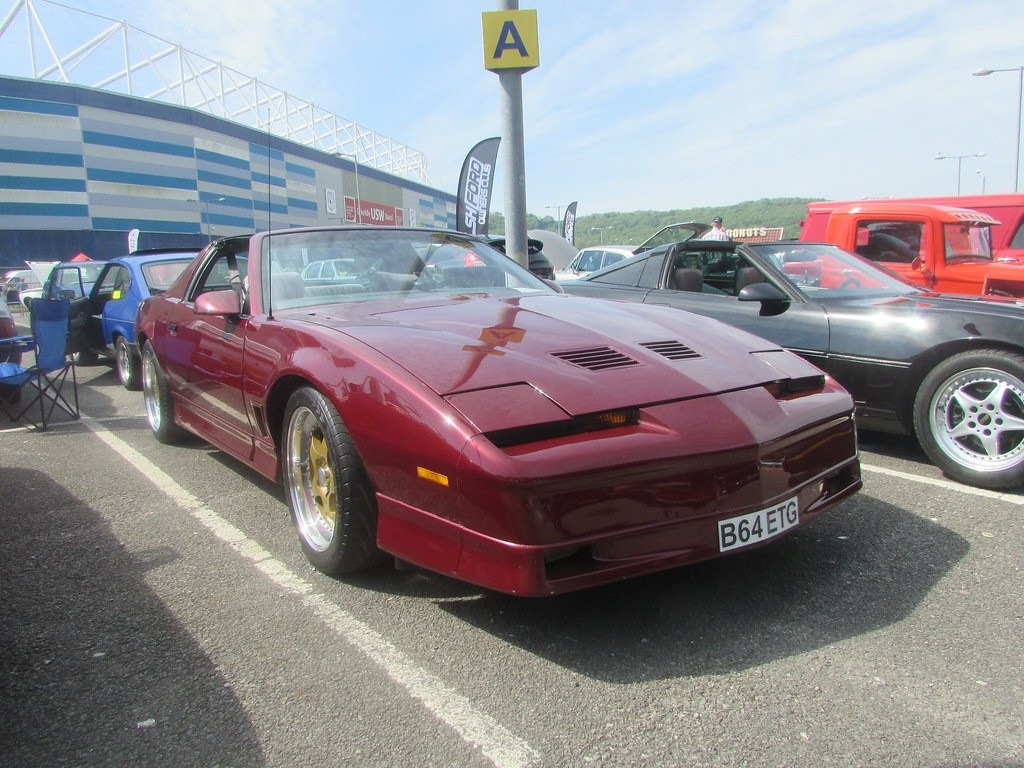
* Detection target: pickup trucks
[821,205,1024,300]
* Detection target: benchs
[301,283,369,300]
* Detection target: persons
[15,277,29,291]
[0,299,18,363]
[698,216,736,274]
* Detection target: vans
[783,193,1024,285]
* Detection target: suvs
[374,229,558,289]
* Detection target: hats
[712,216,722,223]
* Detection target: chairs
[674,268,704,293]
[376,271,419,291]
[0,296,81,433]
[734,267,767,297]
[241,272,307,315]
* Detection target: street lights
[933,152,987,196]
[591,227,614,246]
[972,65,1024,194]
[330,152,362,225]
[544,205,569,236]
[976,170,987,194]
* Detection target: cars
[300,257,359,287]
[0,259,121,313]
[554,220,715,283]
[42,247,249,392]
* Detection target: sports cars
[557,238,1024,491]
[132,225,863,598]
